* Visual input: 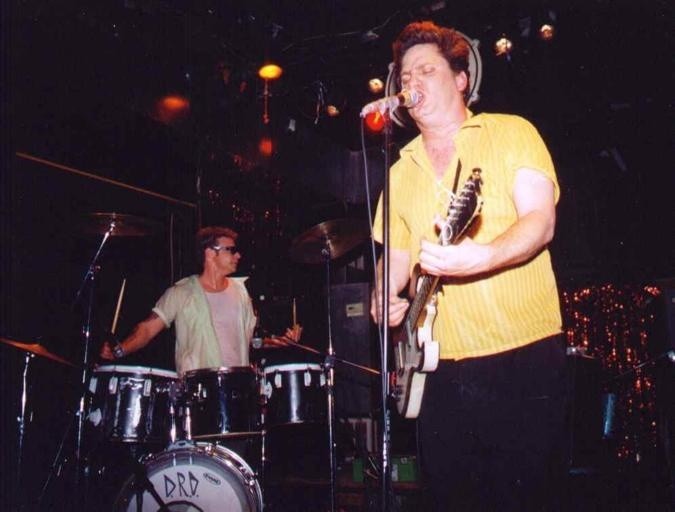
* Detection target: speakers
[332,282,382,418]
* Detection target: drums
[112,440,265,512]
[262,361,329,428]
[181,365,265,439]
[84,365,183,444]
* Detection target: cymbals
[74,212,164,236]
[0,337,77,367]
[289,216,366,264]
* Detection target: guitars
[392,168,484,420]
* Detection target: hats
[385,27,483,133]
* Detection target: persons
[96,226,304,382]
[366,20,572,510]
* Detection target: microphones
[361,88,423,117]
[92,211,136,228]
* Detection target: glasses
[210,245,237,254]
